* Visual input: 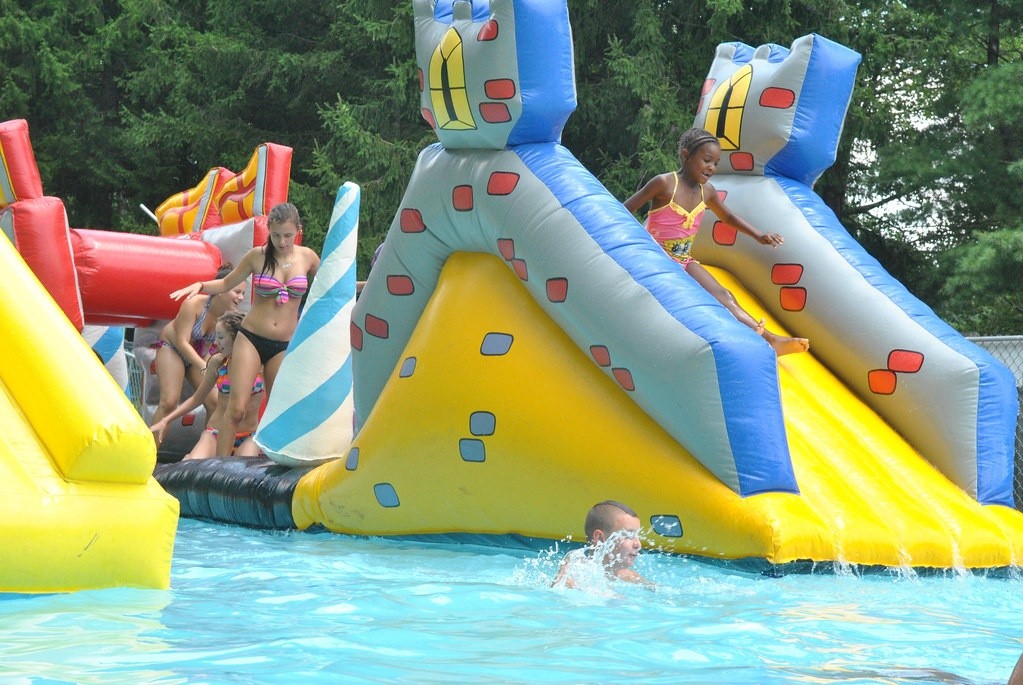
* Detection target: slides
[491,139,1023,582]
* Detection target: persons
[551,500,655,588]
[149,203,367,461]
[622,127,810,358]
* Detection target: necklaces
[213,320,216,324]
[275,253,294,268]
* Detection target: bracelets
[196,281,204,292]
